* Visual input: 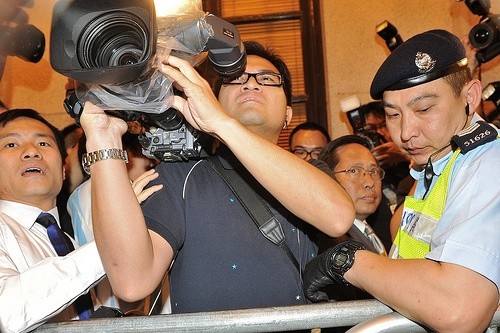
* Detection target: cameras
[375,20,403,53]
[481,80,500,112]
[457,0,500,63]
[339,94,382,152]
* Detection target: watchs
[81,148,130,175]
[329,242,366,288]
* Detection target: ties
[36,213,95,321]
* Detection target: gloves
[302,239,357,302]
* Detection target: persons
[461,33,500,128]
[79,37,356,333]
[301,29,500,333]
[0,100,163,333]
[289,101,421,258]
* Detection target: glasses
[222,70,287,87]
[333,166,386,181]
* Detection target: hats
[370,28,467,100]
